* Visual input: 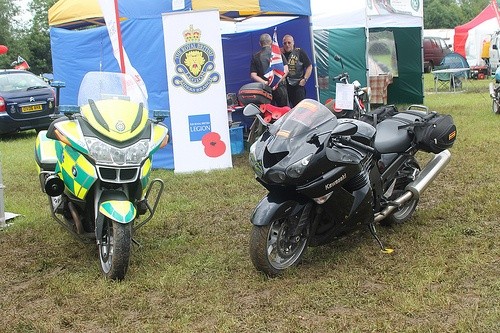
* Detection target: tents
[47,1,321,175]
[311,0,425,109]
[454,0,500,74]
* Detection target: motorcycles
[34,71,170,280]
[238,55,372,151]
[243,99,458,279]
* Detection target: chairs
[433,65,462,91]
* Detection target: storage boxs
[238,81,272,105]
[229,124,245,155]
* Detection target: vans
[391,36,452,73]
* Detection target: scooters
[488,64,500,114]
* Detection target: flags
[263,32,285,90]
[10,56,25,67]
[14,61,30,70]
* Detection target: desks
[432,67,471,93]
[369,71,393,106]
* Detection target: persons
[279,35,312,109]
[250,33,289,108]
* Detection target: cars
[0,69,56,138]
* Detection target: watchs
[303,78,308,82]
